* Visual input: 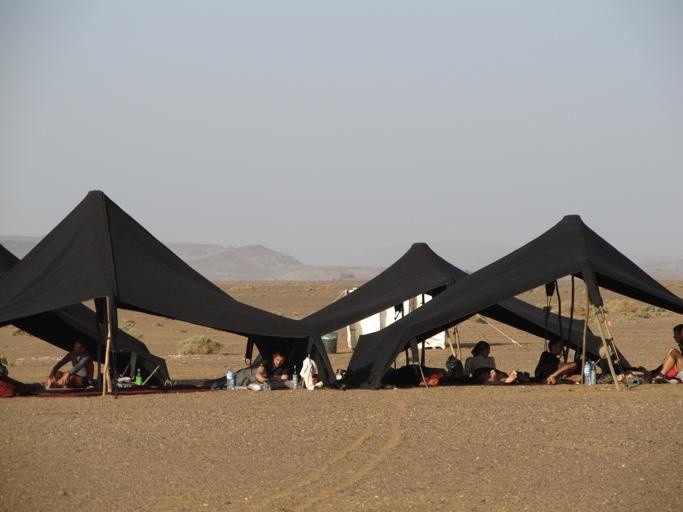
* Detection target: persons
[463,340,518,384]
[206,348,288,389]
[44,338,94,390]
[535,337,581,384]
[649,323,683,384]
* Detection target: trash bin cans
[321,332,337,355]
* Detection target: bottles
[135,368,143,387]
[583,361,592,385]
[226,366,235,391]
[293,373,299,390]
[590,360,597,385]
